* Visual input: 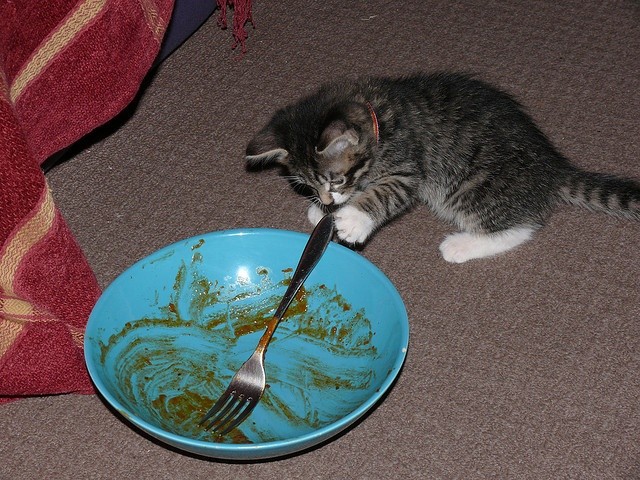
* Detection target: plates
[83,229,410,461]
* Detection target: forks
[200,215,338,436]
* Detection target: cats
[240,67,640,264]
